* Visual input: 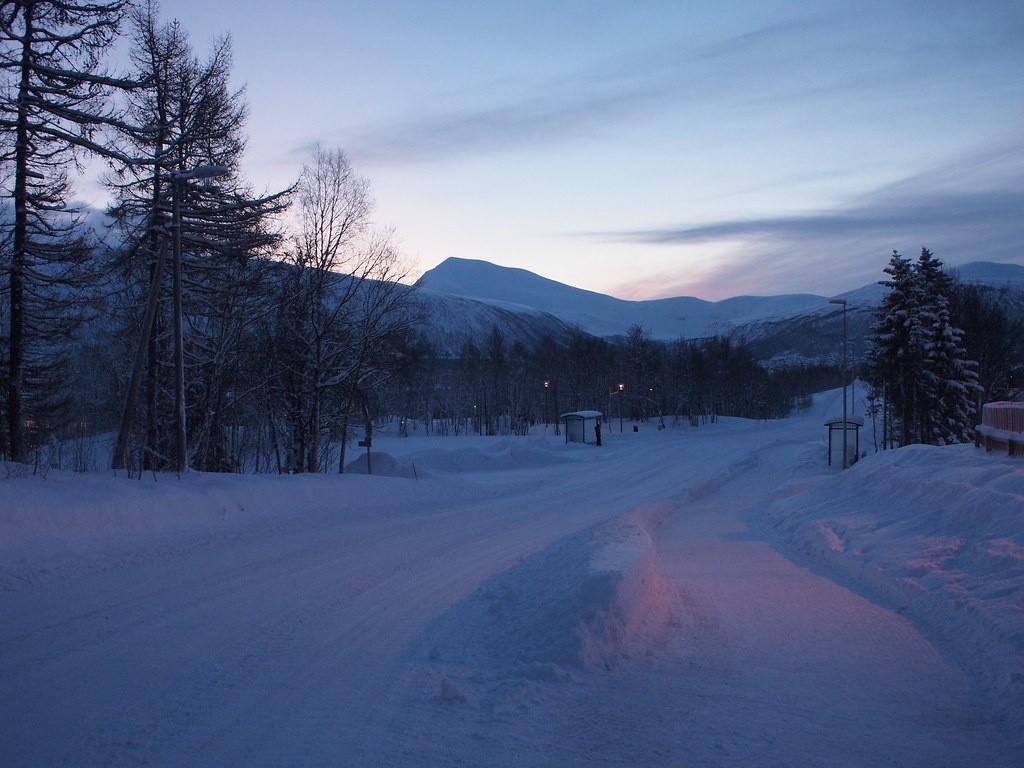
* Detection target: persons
[531,414,536,426]
[595,424,601,446]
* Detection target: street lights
[828,299,847,470]
[543,381,549,428]
[847,342,854,415]
[618,383,624,432]
